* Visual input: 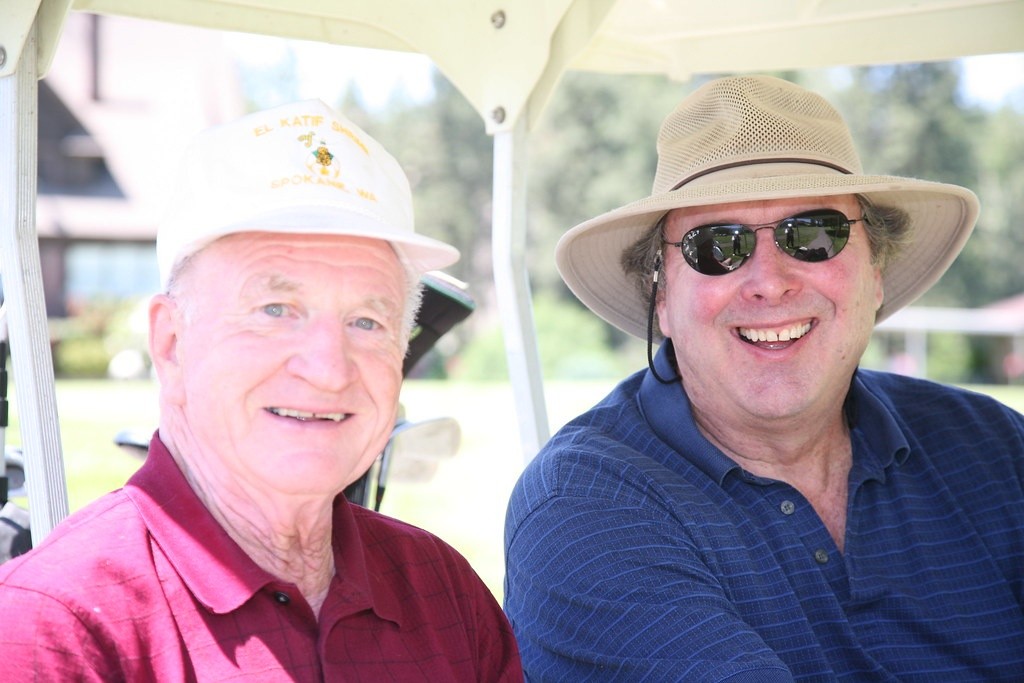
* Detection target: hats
[788,224,792,227]
[556,75,979,345]
[735,231,738,235]
[156,99,460,294]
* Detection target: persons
[501,76,1024,683]
[1,96,525,683]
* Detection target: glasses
[664,209,866,275]
